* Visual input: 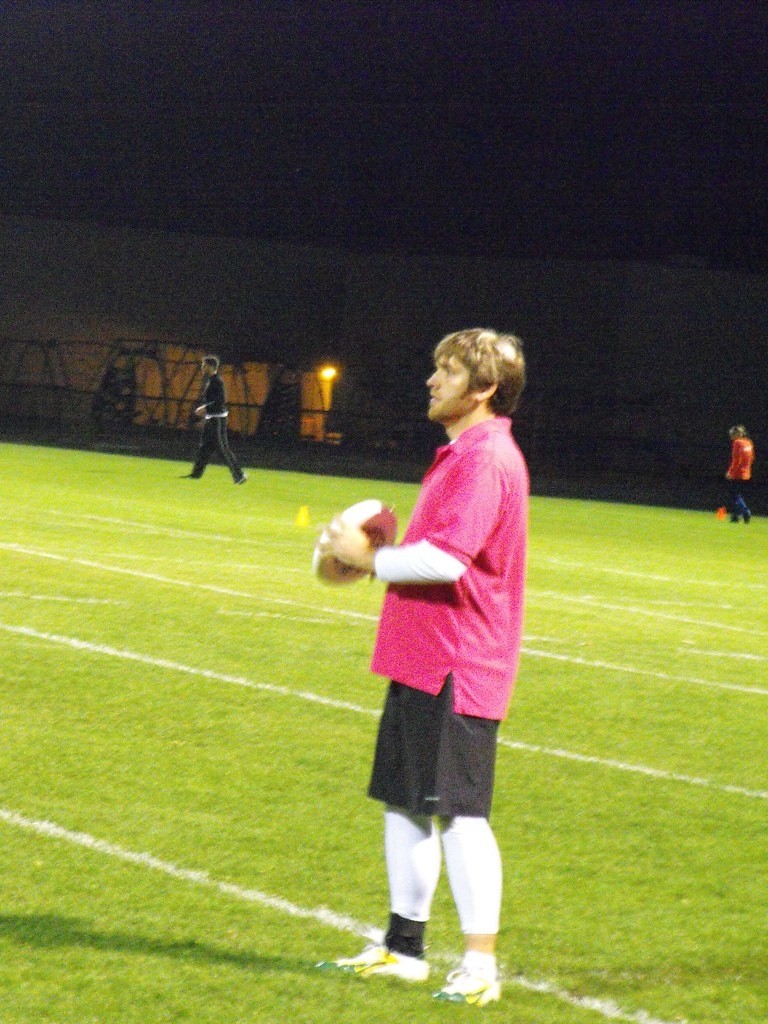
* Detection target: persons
[317,328,528,1009]
[180,355,247,484]
[727,424,755,523]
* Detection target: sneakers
[313,944,429,982]
[432,962,502,1009]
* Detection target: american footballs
[310,496,398,590]
